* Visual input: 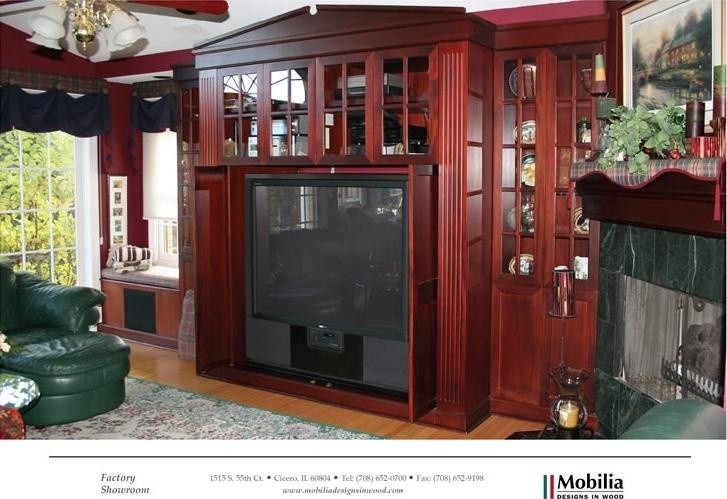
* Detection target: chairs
[0,261,106,370]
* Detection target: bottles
[574,116,596,144]
[686,63,724,158]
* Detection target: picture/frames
[107,173,129,247]
[619,0,727,114]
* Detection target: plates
[573,205,593,237]
[507,62,538,278]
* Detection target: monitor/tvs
[244,179,409,341]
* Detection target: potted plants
[596,97,687,175]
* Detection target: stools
[0,331,130,427]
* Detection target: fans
[0,0,228,16]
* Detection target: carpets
[24,375,390,439]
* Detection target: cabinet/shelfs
[494,13,621,430]
[185,0,493,435]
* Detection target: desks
[505,431,614,440]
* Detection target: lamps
[25,0,147,64]
[538,366,594,439]
[546,269,577,366]
